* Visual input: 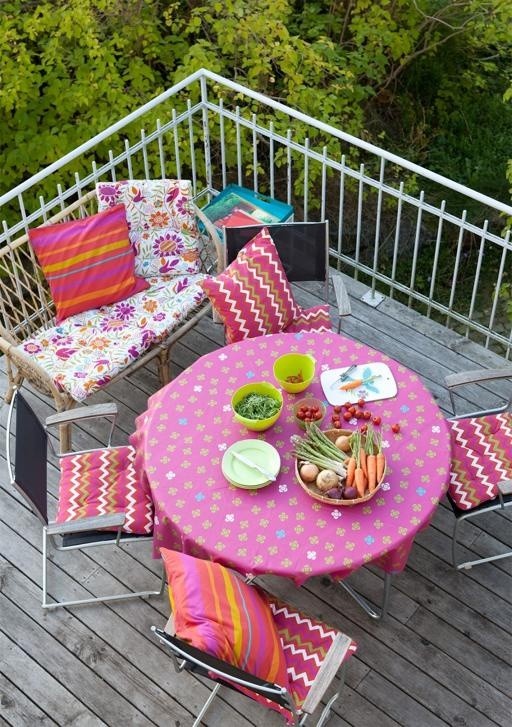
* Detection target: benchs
[0,185,224,461]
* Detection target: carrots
[342,424,386,500]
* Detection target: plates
[222,467,281,489]
[319,361,397,406]
[219,438,282,484]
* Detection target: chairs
[152,587,357,727]
[215,220,354,349]
[446,365,512,573]
[6,382,159,613]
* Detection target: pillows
[159,549,296,716]
[26,204,149,320]
[205,229,299,346]
[94,178,205,277]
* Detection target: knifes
[230,450,278,482]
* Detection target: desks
[185,182,295,277]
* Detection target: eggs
[301,462,320,485]
[334,436,352,451]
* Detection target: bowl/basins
[291,399,326,429]
[272,351,316,392]
[231,381,283,431]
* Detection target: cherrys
[297,404,321,421]
[331,398,400,434]
